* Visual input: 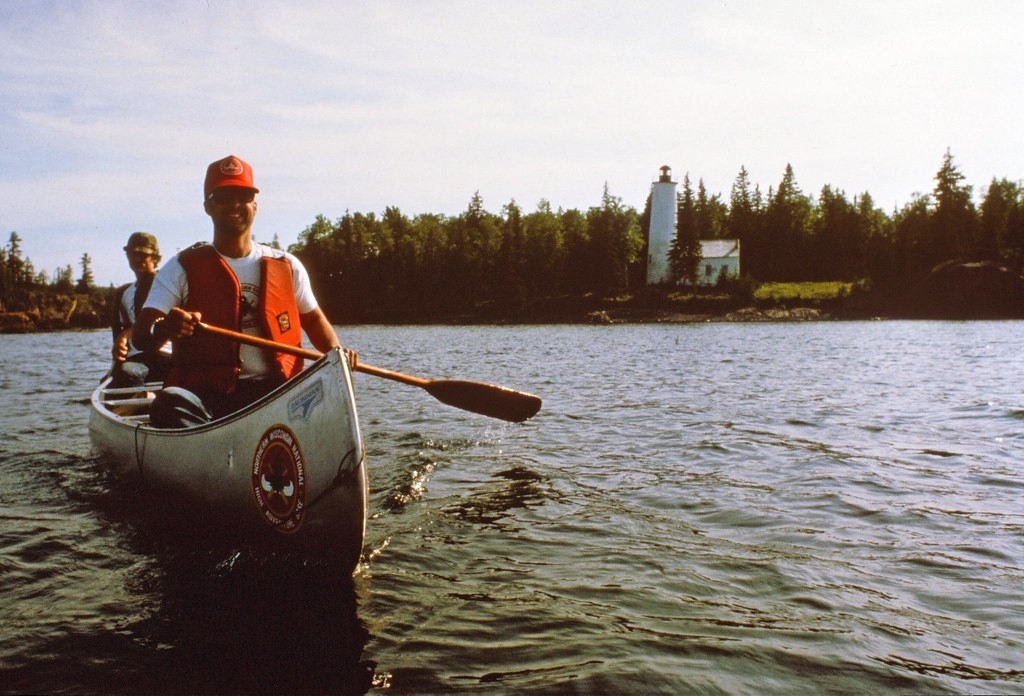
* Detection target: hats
[122,232,160,256]
[204,155,259,202]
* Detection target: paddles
[100,360,120,385]
[195,319,544,425]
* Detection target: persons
[112,233,172,397]
[132,154,358,428]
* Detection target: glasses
[208,188,254,204]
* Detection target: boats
[88,342,372,569]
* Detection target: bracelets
[150,318,163,337]
